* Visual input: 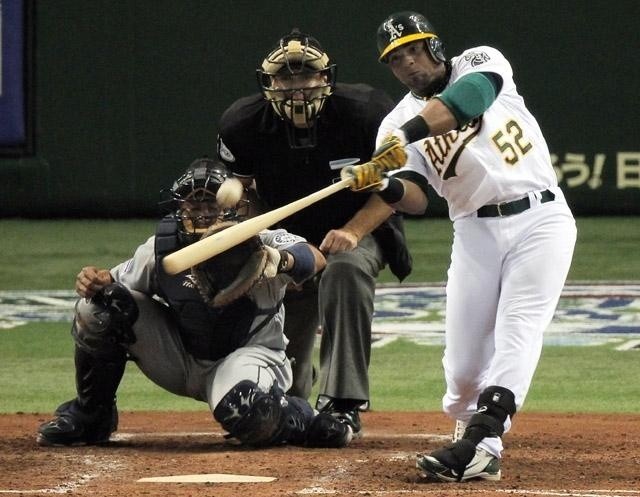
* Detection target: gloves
[340,127,409,192]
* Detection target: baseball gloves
[191,221,267,308]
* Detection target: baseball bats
[162,176,354,276]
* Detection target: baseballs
[216,178,244,208]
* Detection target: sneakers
[417,449,501,481]
[38,416,110,445]
[312,405,362,447]
[452,420,467,442]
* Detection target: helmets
[157,161,253,247]
[256,30,340,163]
[376,12,447,64]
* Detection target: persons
[332,6,580,484]
[214,23,414,432]
[36,157,354,450]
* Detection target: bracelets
[375,171,407,205]
[398,115,430,146]
[277,250,290,273]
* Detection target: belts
[477,191,555,218]
